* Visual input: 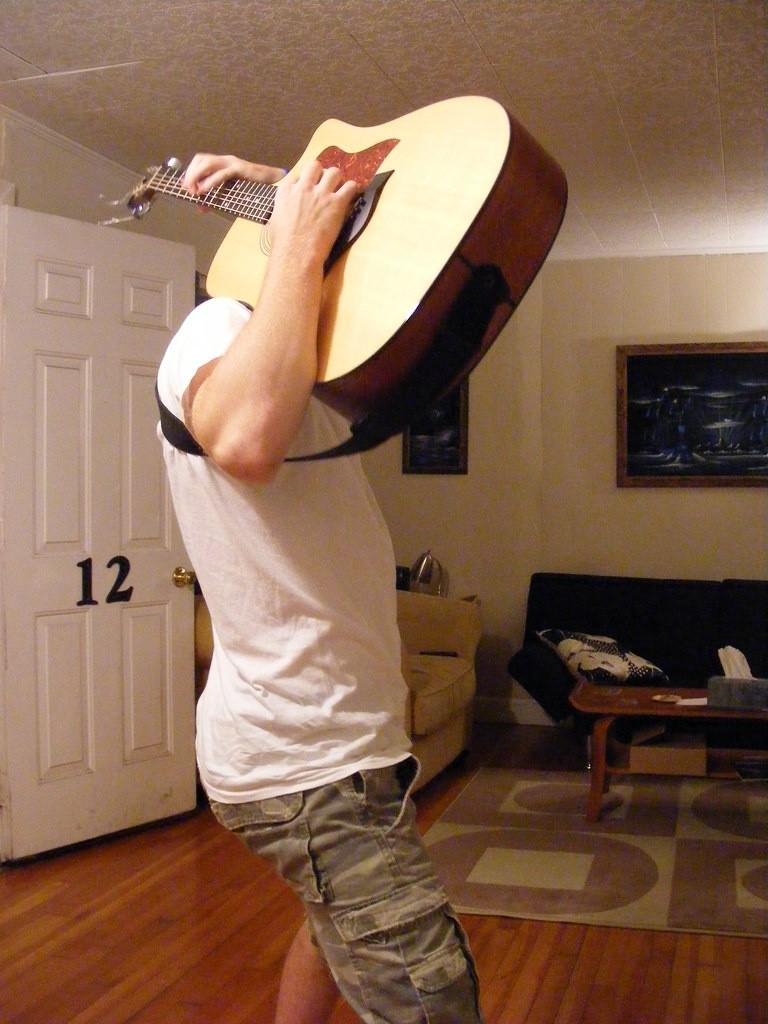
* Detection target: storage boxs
[608,721,707,779]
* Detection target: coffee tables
[567,677,768,822]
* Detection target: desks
[506,573,768,730]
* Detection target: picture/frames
[401,374,467,476]
[615,341,768,487]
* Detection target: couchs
[191,587,479,801]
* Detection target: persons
[155,153,484,1024]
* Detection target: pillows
[535,627,665,685]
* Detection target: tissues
[706,645,768,712]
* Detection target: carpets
[412,765,768,938]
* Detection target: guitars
[125,93,571,430]
